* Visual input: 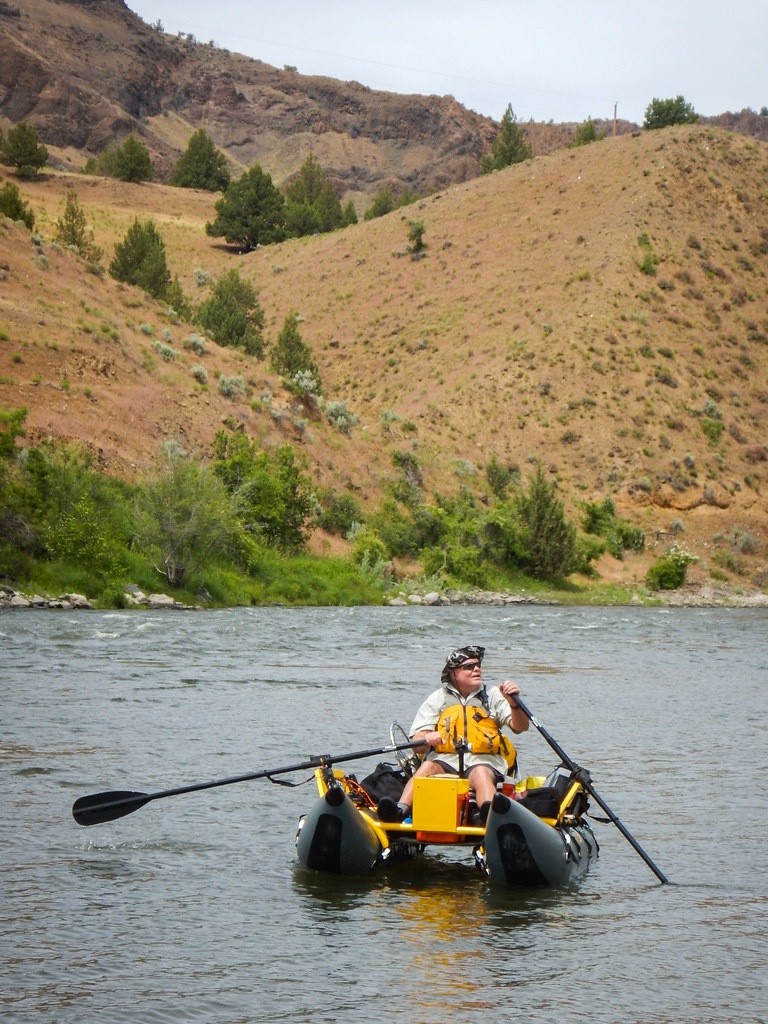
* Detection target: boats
[295,755,600,889]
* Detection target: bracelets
[510,705,519,709]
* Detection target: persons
[377,645,530,840]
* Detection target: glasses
[456,660,481,670]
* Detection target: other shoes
[376,796,407,841]
[478,801,492,827]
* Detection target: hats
[440,644,486,684]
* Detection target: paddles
[72,739,444,827]
[501,684,677,886]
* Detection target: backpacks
[509,787,565,819]
